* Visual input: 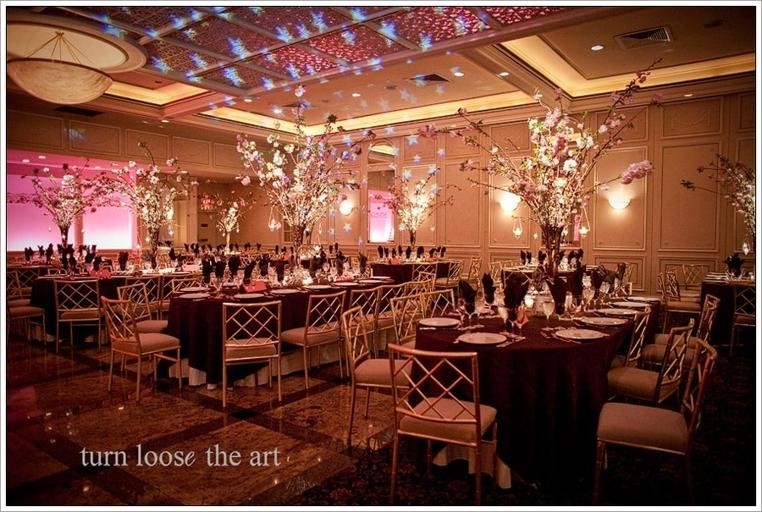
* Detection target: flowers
[7,156,117,226]
[414,53,670,229]
[103,141,197,228]
[187,188,256,237]
[230,85,374,226]
[681,151,756,242]
[371,161,462,230]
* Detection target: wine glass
[463,287,556,340]
[376,246,445,264]
[210,262,376,299]
[559,279,630,313]
[523,255,571,272]
[76,259,204,277]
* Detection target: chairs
[5,248,757,508]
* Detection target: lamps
[7,6,144,106]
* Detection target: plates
[234,275,392,300]
[554,296,663,341]
[460,331,506,345]
[704,268,756,285]
[502,265,523,270]
[418,314,458,327]
[180,284,210,300]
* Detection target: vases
[540,222,561,277]
[147,228,159,253]
[286,220,303,258]
[407,230,415,250]
[61,226,67,248]
